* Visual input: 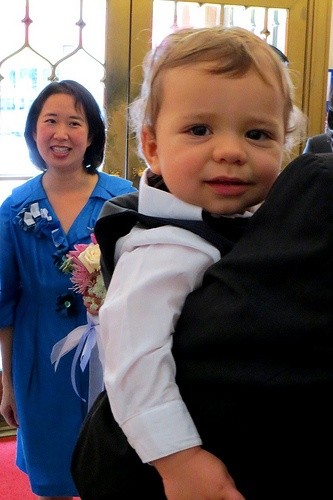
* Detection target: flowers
[60,233,107,317]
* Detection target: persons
[1,79,139,500]
[92,25,303,500]
[69,151,332,500]
